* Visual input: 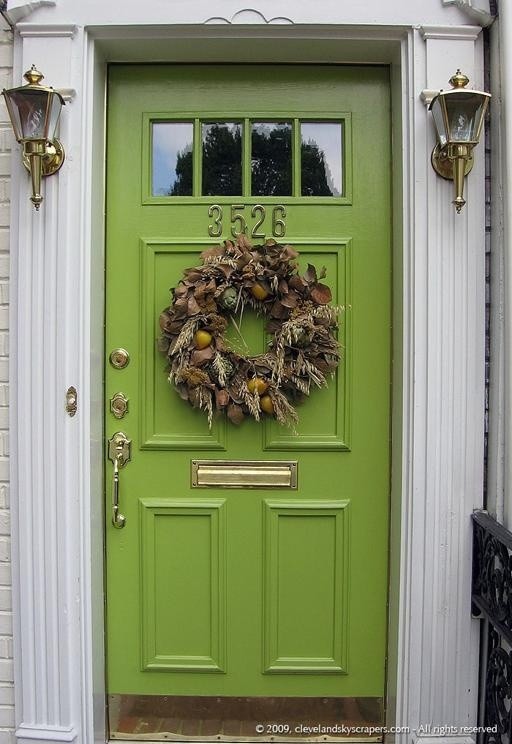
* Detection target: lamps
[427,68,492,214]
[2,64,66,211]
